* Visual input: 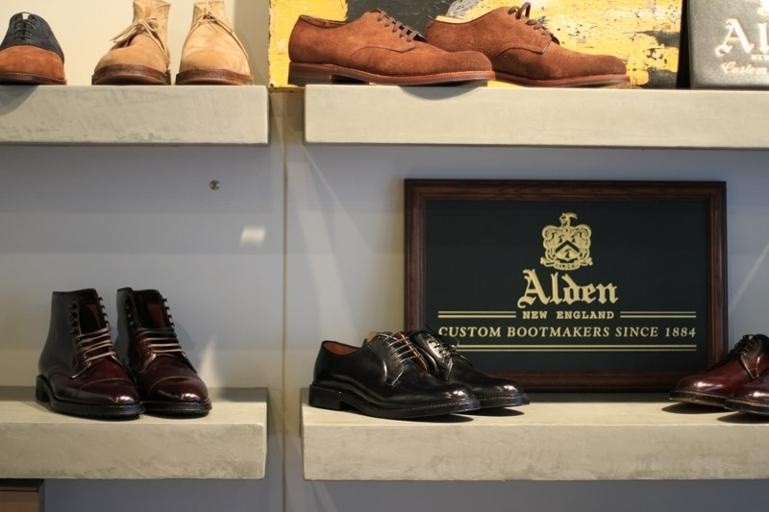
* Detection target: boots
[92,0,173,84]
[176,2,252,85]
[35,286,144,416]
[114,287,211,415]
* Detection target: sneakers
[288,9,495,86]
[426,5,630,86]
[0,12,65,84]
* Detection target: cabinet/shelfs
[0,81,768,512]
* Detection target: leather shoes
[307,327,530,419]
[669,333,769,415]
